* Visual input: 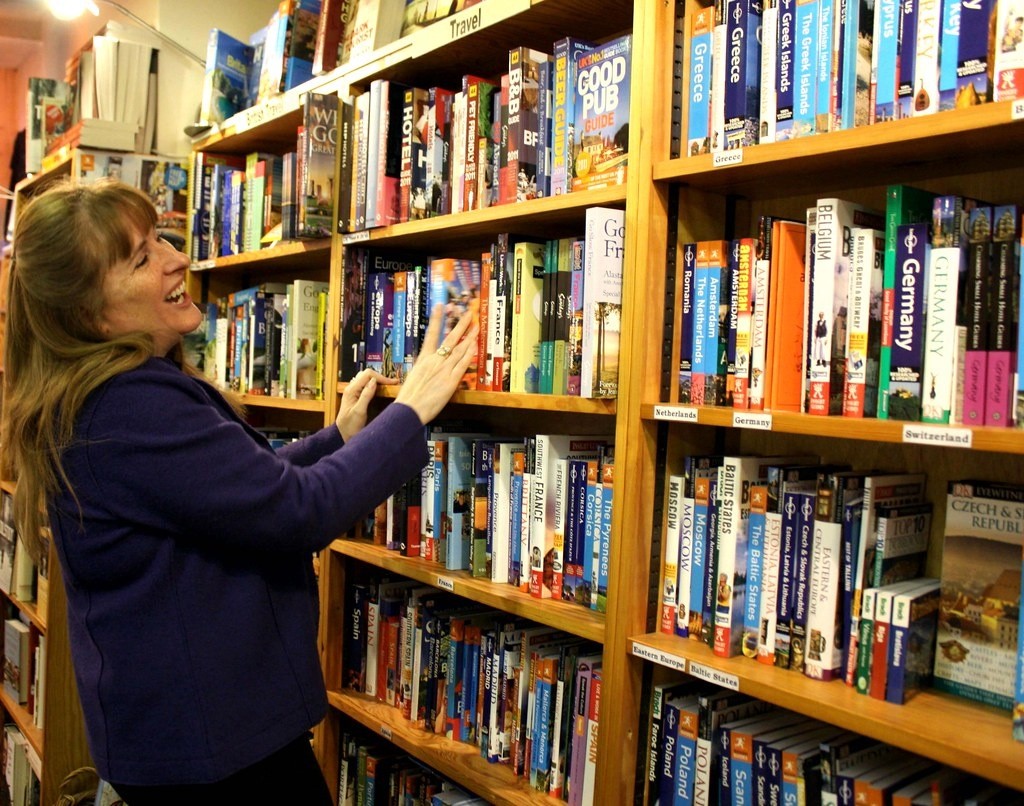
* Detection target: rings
[437,345,452,357]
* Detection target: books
[334,27,632,806]
[201,0,474,127]
[633,0,1023,806]
[117,91,338,401]
[1,18,162,804]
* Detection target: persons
[0,174,481,806]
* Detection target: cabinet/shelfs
[1,148,201,805]
[313,0,646,806]
[177,62,349,805]
[606,1,1023,806]
